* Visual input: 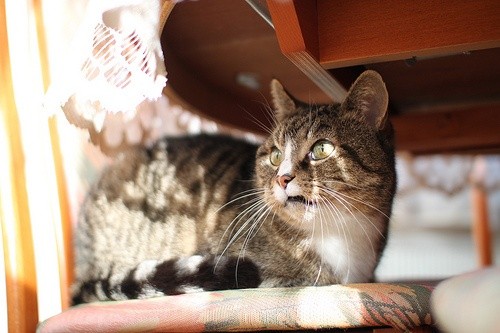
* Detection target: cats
[67,69,396,307]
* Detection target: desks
[156,0,500,156]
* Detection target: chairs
[0,0,438,333]
